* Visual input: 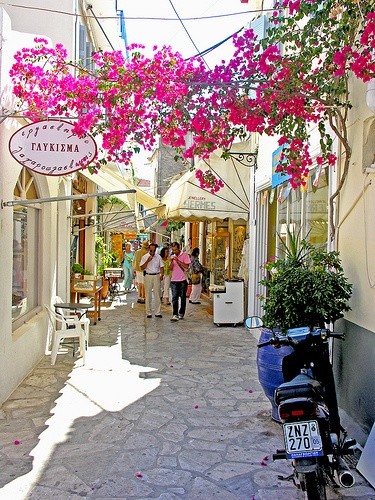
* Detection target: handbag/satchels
[191,274,200,284]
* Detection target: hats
[140,240,149,248]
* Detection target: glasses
[150,250,156,252]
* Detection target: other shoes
[167,303,171,305]
[192,302,201,304]
[127,290,131,293]
[147,315,152,318]
[178,313,183,319]
[124,290,128,294]
[140,299,145,304]
[161,298,164,303]
[189,300,192,303]
[155,314,162,318]
[137,299,141,303]
[170,314,179,321]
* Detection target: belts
[146,273,159,275]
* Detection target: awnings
[72,161,166,232]
[160,142,250,222]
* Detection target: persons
[155,242,172,256]
[183,238,192,253]
[189,248,204,304]
[169,242,191,321]
[140,243,164,318]
[133,241,148,304]
[121,241,138,291]
[160,247,172,305]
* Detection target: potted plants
[256,221,353,423]
[72,264,92,280]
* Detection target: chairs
[44,295,90,366]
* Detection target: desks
[54,303,93,357]
[103,267,122,303]
[71,274,103,326]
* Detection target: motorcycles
[245,316,357,500]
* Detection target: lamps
[223,134,258,169]
